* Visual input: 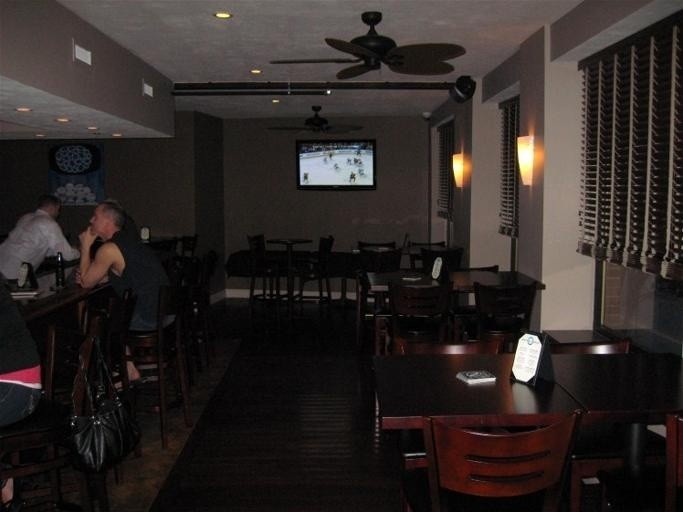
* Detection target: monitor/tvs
[296,138,375,190]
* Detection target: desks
[359,245,452,269]
[447,270,546,323]
[536,352,683,511]
[268,236,314,321]
[363,269,442,342]
[11,274,117,401]
[369,353,587,499]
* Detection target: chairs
[394,332,505,355]
[295,234,337,304]
[359,248,402,319]
[475,281,538,314]
[422,412,579,512]
[540,338,635,354]
[388,281,453,314]
[127,273,192,448]
[359,240,398,251]
[169,258,201,385]
[179,233,200,256]
[93,289,143,487]
[248,235,287,302]
[194,252,219,372]
[0,312,107,512]
[411,241,447,246]
[668,413,683,512]
[421,247,464,279]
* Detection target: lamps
[451,154,463,188]
[517,135,534,187]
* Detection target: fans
[269,12,466,80]
[267,106,365,135]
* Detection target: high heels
[2,463,26,511]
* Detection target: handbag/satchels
[68,337,141,473]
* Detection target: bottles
[56,251,64,288]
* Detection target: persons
[0,194,80,279]
[78,199,171,413]
[0,273,43,512]
